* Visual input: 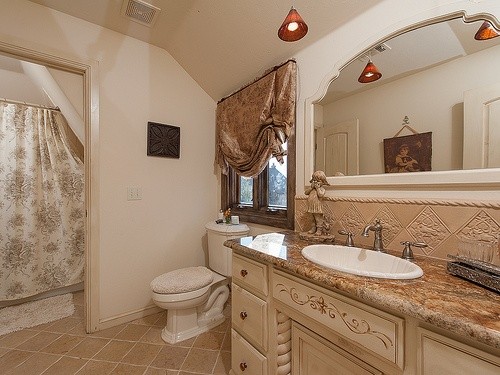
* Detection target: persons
[305,170,331,236]
[390,143,419,173]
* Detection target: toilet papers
[231,214,240,224]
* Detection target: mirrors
[303,9,499,188]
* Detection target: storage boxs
[444,252,500,294]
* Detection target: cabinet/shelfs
[220,241,500,375]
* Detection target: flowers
[225,207,232,224]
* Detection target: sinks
[299,243,429,279]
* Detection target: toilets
[149,223,251,345]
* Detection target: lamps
[472,19,500,40]
[356,50,383,84]
[275,0,310,43]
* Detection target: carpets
[0,292,76,336]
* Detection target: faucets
[364,218,389,250]
